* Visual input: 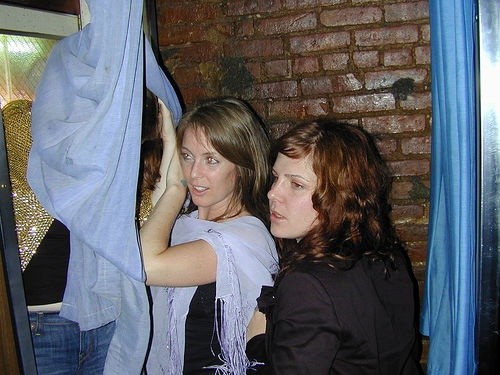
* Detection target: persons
[137,95,279,375]
[246,119,425,375]
[0,88,164,375]
